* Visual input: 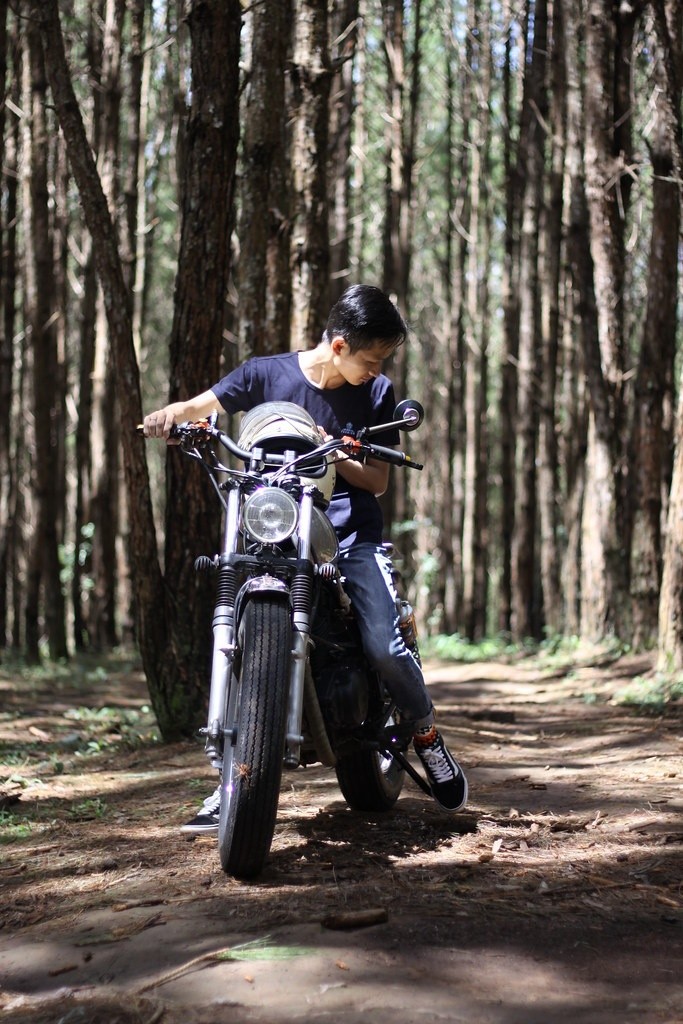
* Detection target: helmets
[237,403,337,512]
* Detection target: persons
[144,284,468,833]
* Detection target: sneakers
[413,731,468,814]
[180,785,221,835]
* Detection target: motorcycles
[134,397,425,882]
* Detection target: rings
[151,418,157,420]
